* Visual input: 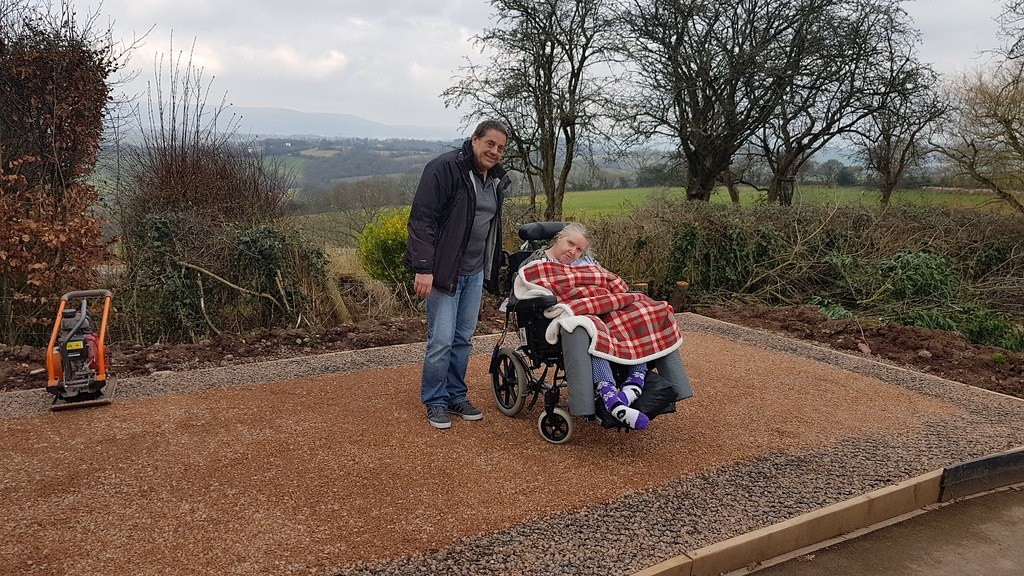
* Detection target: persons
[507,222,650,430]
[403,120,512,428]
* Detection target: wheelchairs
[487,222,676,446]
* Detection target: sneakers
[426,404,451,428]
[447,400,483,420]
